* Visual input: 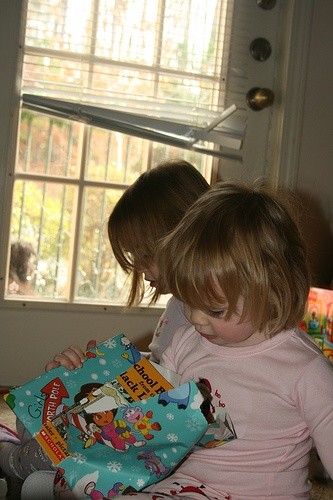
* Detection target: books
[33,354,176,468]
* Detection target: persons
[0,159,213,482]
[43,184,333,500]
[7,240,39,298]
